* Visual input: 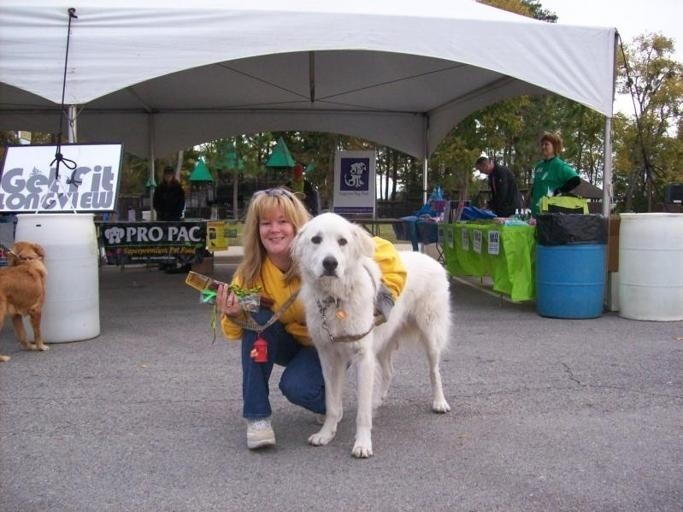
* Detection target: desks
[94,217,246,273]
[346,218,540,309]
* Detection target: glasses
[253,186,295,208]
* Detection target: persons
[476,157,523,217]
[154,166,184,270]
[526,133,580,225]
[215,188,407,449]
[282,161,315,212]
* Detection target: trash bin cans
[535,214,609,319]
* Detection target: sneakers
[246,417,276,449]
[316,398,343,424]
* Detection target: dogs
[288,212,452,458]
[0,241,50,363]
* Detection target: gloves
[375,295,394,325]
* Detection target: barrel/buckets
[15,213,101,343]
[618,212,683,322]
